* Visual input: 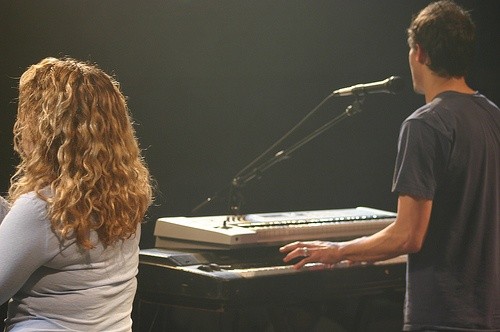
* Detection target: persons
[279,1,499,331]
[0,57,152,331]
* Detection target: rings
[303,246,308,257]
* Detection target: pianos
[132,206,410,331]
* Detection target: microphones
[333,76,405,98]
